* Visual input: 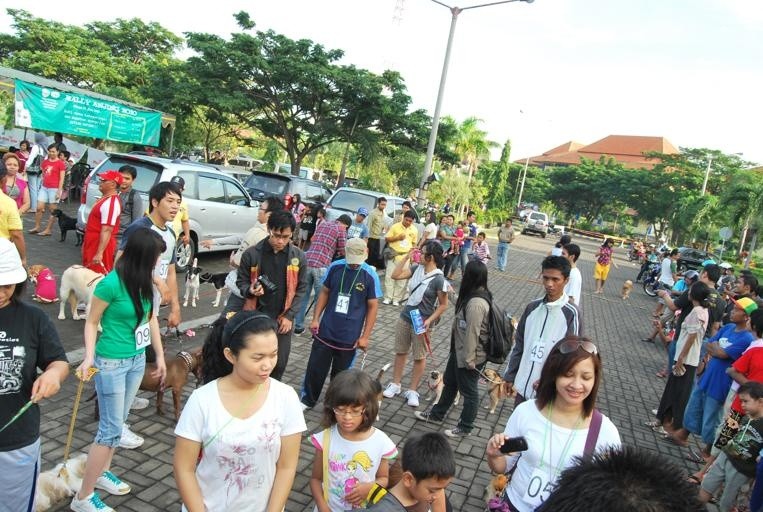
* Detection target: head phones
[425,242,438,262]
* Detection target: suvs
[239,168,332,242]
[72,150,261,273]
[520,211,549,238]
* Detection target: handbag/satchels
[433,305,441,326]
[462,293,513,364]
[485,498,510,512]
[26,155,45,175]
[384,247,397,259]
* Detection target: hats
[96,171,123,185]
[0,237,27,286]
[730,296,757,316]
[170,176,185,188]
[358,208,369,216]
[346,238,367,265]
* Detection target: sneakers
[405,391,419,406]
[120,424,144,449]
[383,299,391,304]
[383,383,402,398]
[28,228,40,233]
[652,410,657,414]
[38,231,52,236]
[131,398,149,410]
[444,428,471,437]
[415,410,444,426]
[392,302,399,306]
[96,471,131,496]
[70,491,115,512]
[294,328,305,337]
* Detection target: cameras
[254,274,280,295]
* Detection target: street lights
[513,107,532,218]
[676,143,744,249]
[417,0,531,215]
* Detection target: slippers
[642,337,655,343]
[685,451,713,463]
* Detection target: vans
[315,185,423,270]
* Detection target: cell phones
[500,436,528,454]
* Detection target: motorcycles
[547,223,563,239]
[643,262,689,297]
[627,245,646,265]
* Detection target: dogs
[622,279,633,300]
[479,368,517,415]
[33,453,89,512]
[425,370,461,406]
[27,264,105,333]
[183,258,230,308]
[371,362,393,422]
[484,474,510,503]
[84,350,203,423]
[51,209,84,247]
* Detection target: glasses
[269,229,293,240]
[557,341,597,357]
[333,407,365,416]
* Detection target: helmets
[702,260,715,267]
[720,262,732,274]
[683,270,698,283]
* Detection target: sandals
[685,473,702,484]
[646,420,689,447]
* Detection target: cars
[712,247,727,254]
[127,140,358,192]
[670,246,723,276]
